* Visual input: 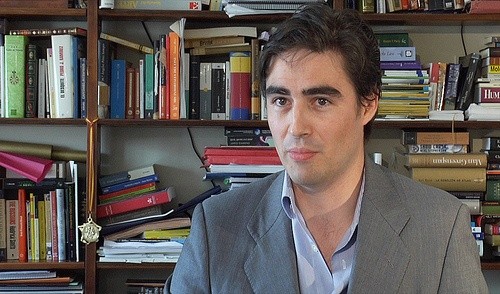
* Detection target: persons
[163,3,490,294]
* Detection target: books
[0,0,500,120]
[0,129,500,294]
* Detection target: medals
[79,218,102,244]
